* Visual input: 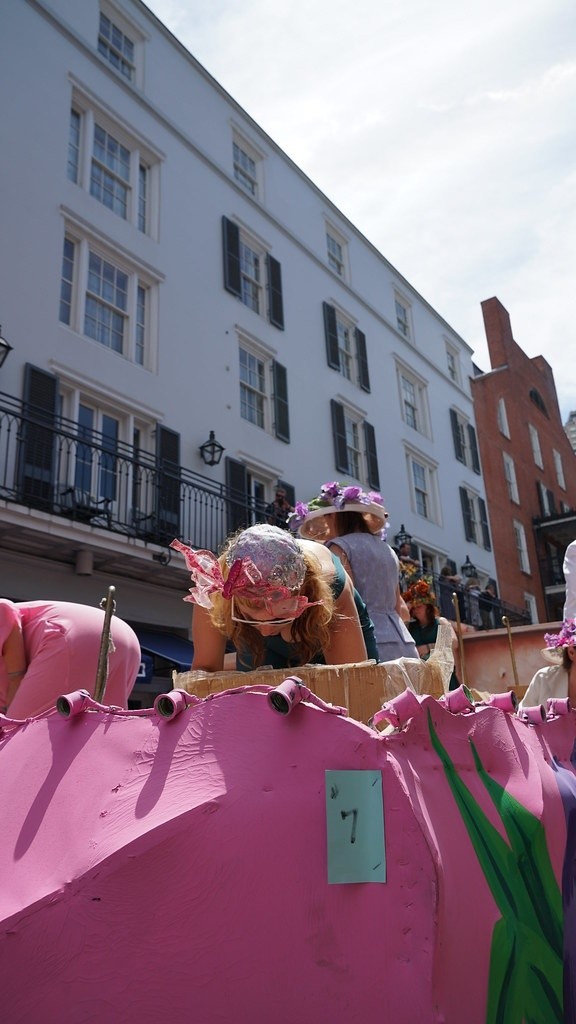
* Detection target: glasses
[231,594,295,627]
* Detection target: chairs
[57,484,113,529]
[131,508,184,545]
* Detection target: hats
[287,481,388,542]
[541,619,576,665]
[168,522,307,610]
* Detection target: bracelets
[287,505,291,510]
[427,643,431,654]
[8,670,26,677]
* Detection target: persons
[439,565,496,627]
[518,618,576,710]
[401,579,440,661]
[398,543,421,592]
[265,489,295,530]
[170,525,380,671]
[563,540,576,622]
[0,599,141,730]
[286,482,420,661]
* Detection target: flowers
[401,575,435,603]
[286,481,383,523]
[544,616,576,654]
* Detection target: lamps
[461,555,476,578]
[394,524,412,546]
[199,431,226,466]
